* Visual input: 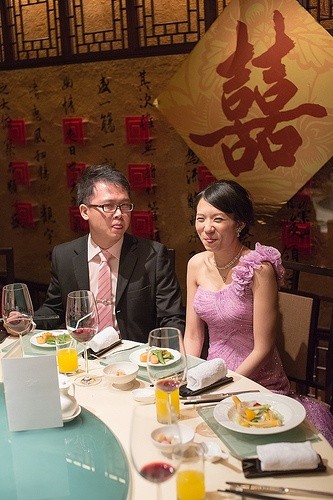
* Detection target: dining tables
[0,329,333,500]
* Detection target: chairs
[275,288,320,394]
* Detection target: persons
[3,164,185,352]
[183,181,333,448]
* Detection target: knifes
[180,390,259,400]
[225,482,333,500]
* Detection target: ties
[92,251,115,333]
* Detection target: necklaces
[211,244,245,283]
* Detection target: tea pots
[58,377,77,417]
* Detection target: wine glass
[66,290,102,386]
[130,403,184,500]
[147,328,187,425]
[2,283,34,358]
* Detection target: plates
[130,389,155,402]
[128,346,181,367]
[212,392,306,436]
[29,330,73,347]
[63,406,81,423]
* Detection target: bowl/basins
[150,424,195,453]
[102,362,139,385]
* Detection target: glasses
[87,202,135,213]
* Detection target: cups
[172,443,205,500]
[55,337,79,376]
[153,370,180,423]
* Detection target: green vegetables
[152,349,174,364]
[251,405,270,421]
[47,334,71,344]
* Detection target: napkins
[186,358,228,391]
[256,441,320,471]
[90,326,119,353]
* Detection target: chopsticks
[217,489,286,500]
[184,399,223,405]
